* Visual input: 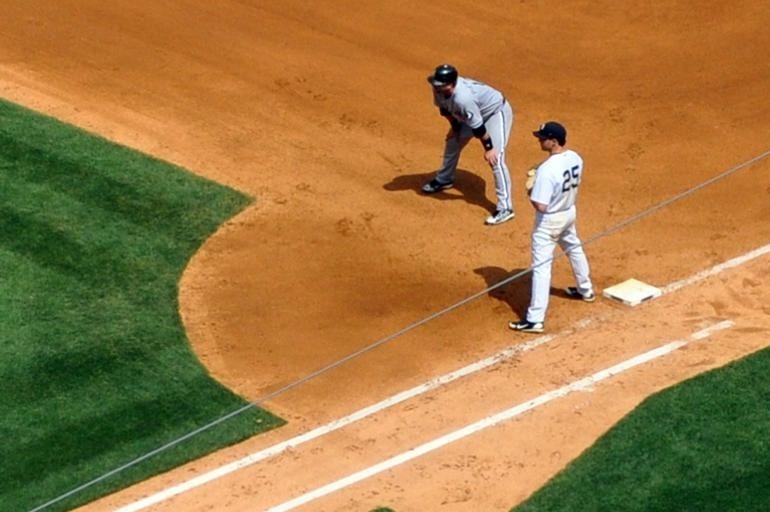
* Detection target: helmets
[428,64,457,87]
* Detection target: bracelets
[482,137,493,151]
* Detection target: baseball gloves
[525,167,539,195]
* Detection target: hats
[533,122,566,140]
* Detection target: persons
[421,63,515,225]
[509,120,596,333]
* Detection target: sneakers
[509,319,544,333]
[566,286,596,302]
[485,209,515,224]
[422,178,455,192]
